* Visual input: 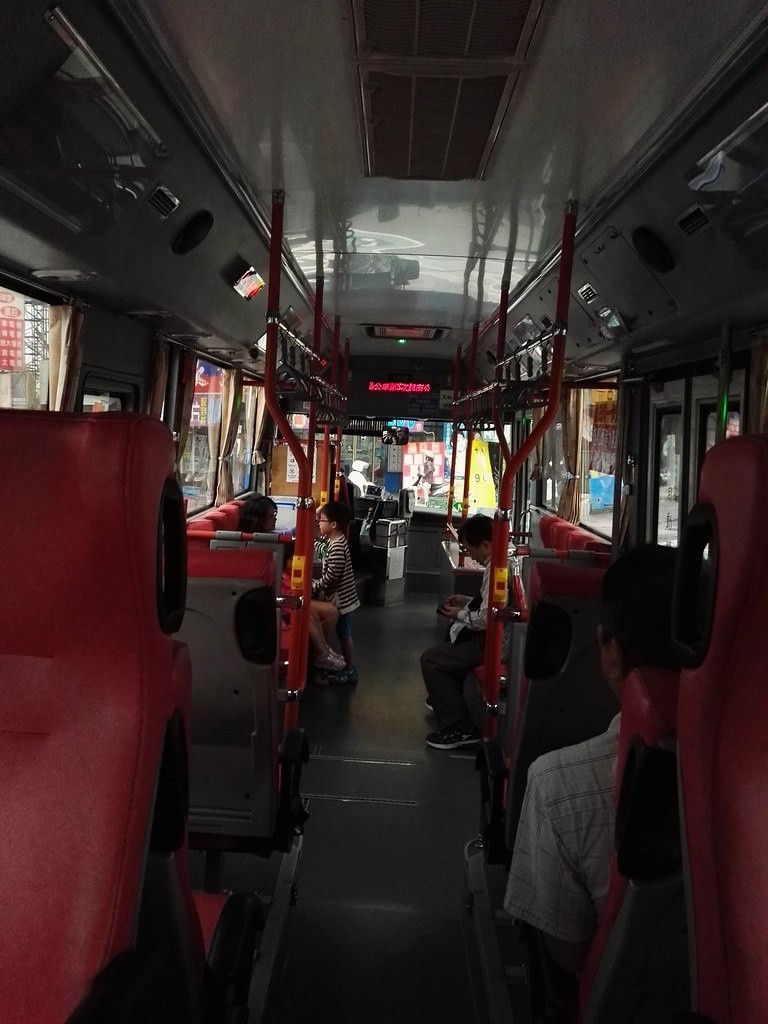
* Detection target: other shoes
[322,670,353,682]
[312,649,346,671]
[325,646,345,661]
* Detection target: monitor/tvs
[366,485,386,499]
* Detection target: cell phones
[439,604,450,612]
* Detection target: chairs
[473,431,768,1024]
[0,404,291,1024]
[330,464,356,543]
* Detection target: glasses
[268,510,278,517]
[318,516,333,523]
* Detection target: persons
[310,501,361,684]
[503,544,714,972]
[447,431,482,479]
[383,428,408,444]
[348,460,368,498]
[420,514,530,749]
[329,444,361,537]
[237,494,346,670]
[417,451,435,507]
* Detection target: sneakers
[425,719,483,749]
[424,696,435,712]
